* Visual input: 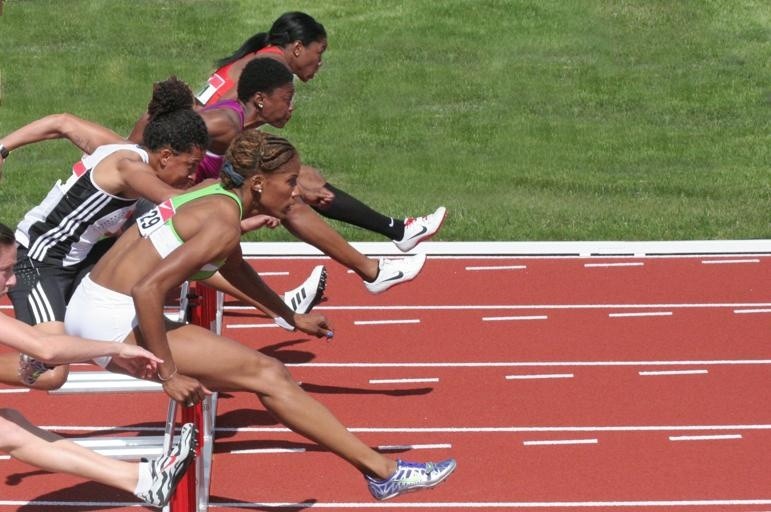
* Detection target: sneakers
[362,253,427,295]
[392,206,448,253]
[137,422,199,509]
[364,458,457,501]
[17,351,55,387]
[274,265,328,333]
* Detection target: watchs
[0,143,10,160]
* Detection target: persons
[0,77,328,389]
[16,133,458,502]
[0,224,199,510]
[126,55,427,295]
[192,12,450,253]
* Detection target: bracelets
[156,367,178,383]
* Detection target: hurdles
[161,280,223,512]
